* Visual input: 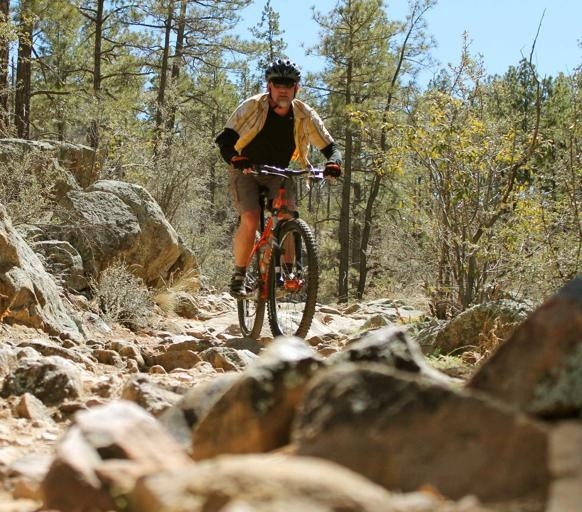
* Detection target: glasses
[270,78,297,88]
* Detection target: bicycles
[234,160,328,346]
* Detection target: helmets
[265,60,301,82]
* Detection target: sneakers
[279,272,304,289]
[229,273,249,300]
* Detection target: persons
[214,59,342,299]
